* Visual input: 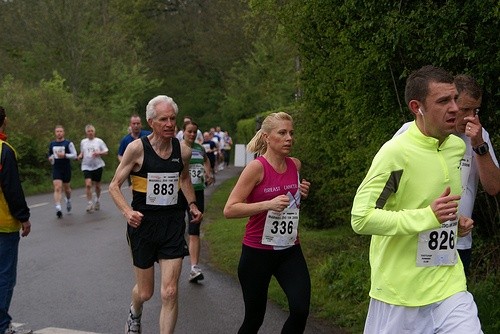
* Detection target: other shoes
[56,209,63,218]
[93,198,100,210]
[86,203,93,213]
[65,199,72,212]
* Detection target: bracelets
[188,201,197,207]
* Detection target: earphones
[418,108,423,115]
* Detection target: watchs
[473,141,489,155]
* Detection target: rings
[135,222,137,225]
[469,127,470,131]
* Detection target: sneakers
[4,322,33,334]
[124,303,142,334]
[188,268,204,283]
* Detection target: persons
[46,124,77,218]
[200,132,218,182]
[0,106,31,334]
[127,126,134,133]
[223,112,311,334]
[209,128,221,174]
[108,95,204,334]
[215,125,225,170]
[176,117,204,142]
[116,113,151,191]
[223,130,232,169]
[350,64,487,334]
[392,74,500,278]
[179,121,213,283]
[77,124,108,213]
